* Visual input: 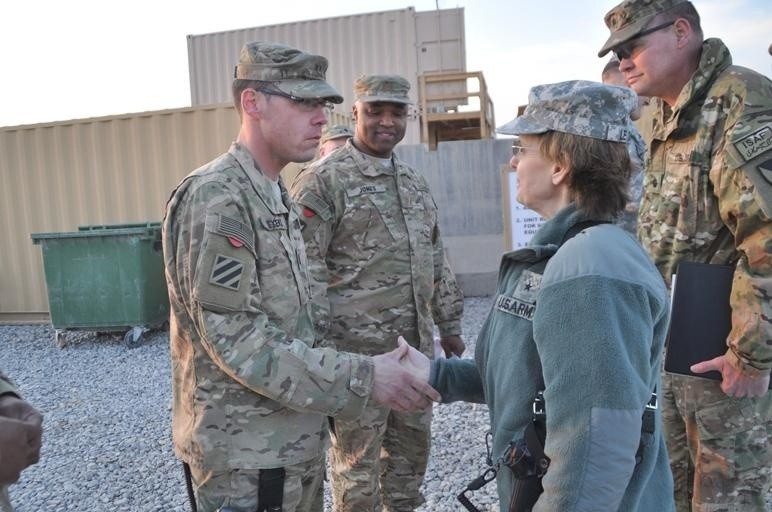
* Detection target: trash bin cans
[30,222,170,349]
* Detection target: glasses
[256,84,335,114]
[511,138,545,158]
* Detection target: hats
[234,42,345,106]
[354,74,415,107]
[501,79,647,161]
[599,1,699,56]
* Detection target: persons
[0,372,44,512]
[601,53,653,239]
[281,73,464,512]
[397,80,677,511]
[159,41,443,512]
[597,0,772,511]
[318,126,353,157]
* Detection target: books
[662,261,772,390]
